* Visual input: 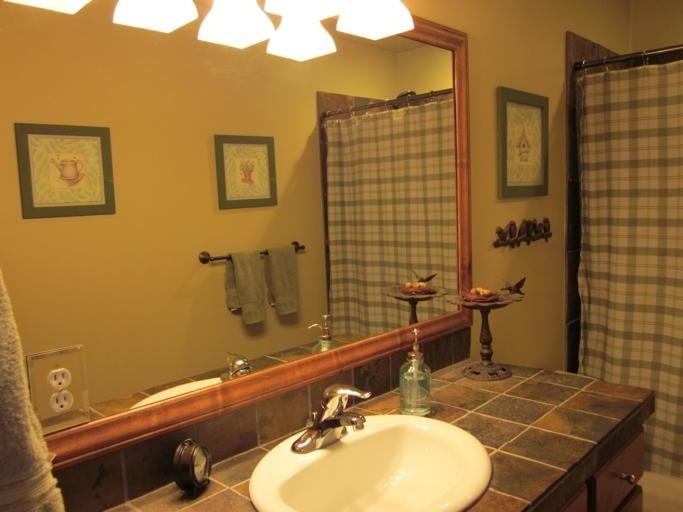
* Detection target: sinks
[128,376,223,410]
[249,414,492,512]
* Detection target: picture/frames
[214,133,277,209]
[14,123,115,219]
[496,86,550,198]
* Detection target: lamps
[336,0,415,42]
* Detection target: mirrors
[0,0,474,470]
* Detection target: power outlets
[26,345,87,420]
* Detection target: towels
[0,267,65,511]
[225,252,270,326]
[262,245,297,315]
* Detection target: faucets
[219,352,250,382]
[289,384,372,452]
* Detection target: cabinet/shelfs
[560,426,646,512]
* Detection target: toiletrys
[309,314,336,354]
[398,328,431,416]
